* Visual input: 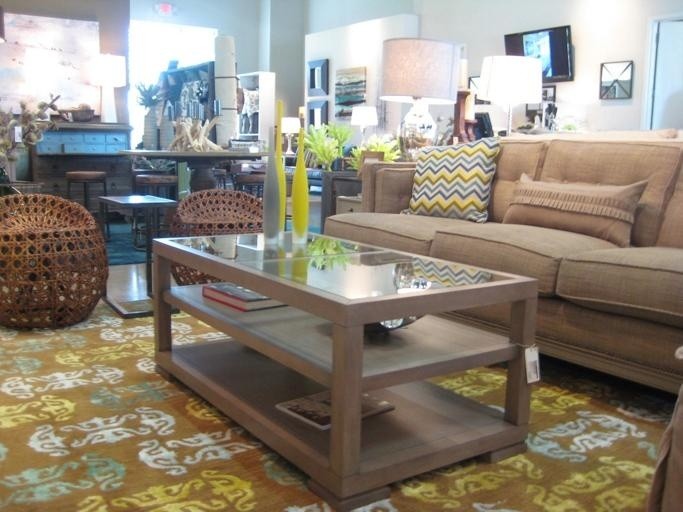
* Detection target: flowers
[0,96,61,168]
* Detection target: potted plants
[347,142,399,176]
[303,126,339,173]
[322,122,358,157]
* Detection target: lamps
[378,36,459,148]
[476,55,543,137]
[281,117,301,155]
[351,106,378,133]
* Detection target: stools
[1,194,108,329]
[66,171,111,244]
[238,172,295,220]
[169,190,263,285]
[134,173,178,247]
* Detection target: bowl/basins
[71,109,95,123]
[58,110,74,123]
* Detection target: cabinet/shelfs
[321,171,362,233]
[29,123,135,224]
[158,62,217,143]
[236,72,277,140]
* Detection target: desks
[122,149,263,201]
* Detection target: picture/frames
[305,101,328,126]
[308,59,328,97]
[468,76,492,106]
[333,66,367,125]
[599,61,633,99]
[525,84,556,117]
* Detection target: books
[203,282,288,312]
[276,390,395,430]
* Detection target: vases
[0,174,22,198]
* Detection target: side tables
[99,195,181,319]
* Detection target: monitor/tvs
[504,24,574,82]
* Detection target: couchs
[323,129,683,397]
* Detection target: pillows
[502,170,648,250]
[399,138,503,223]
